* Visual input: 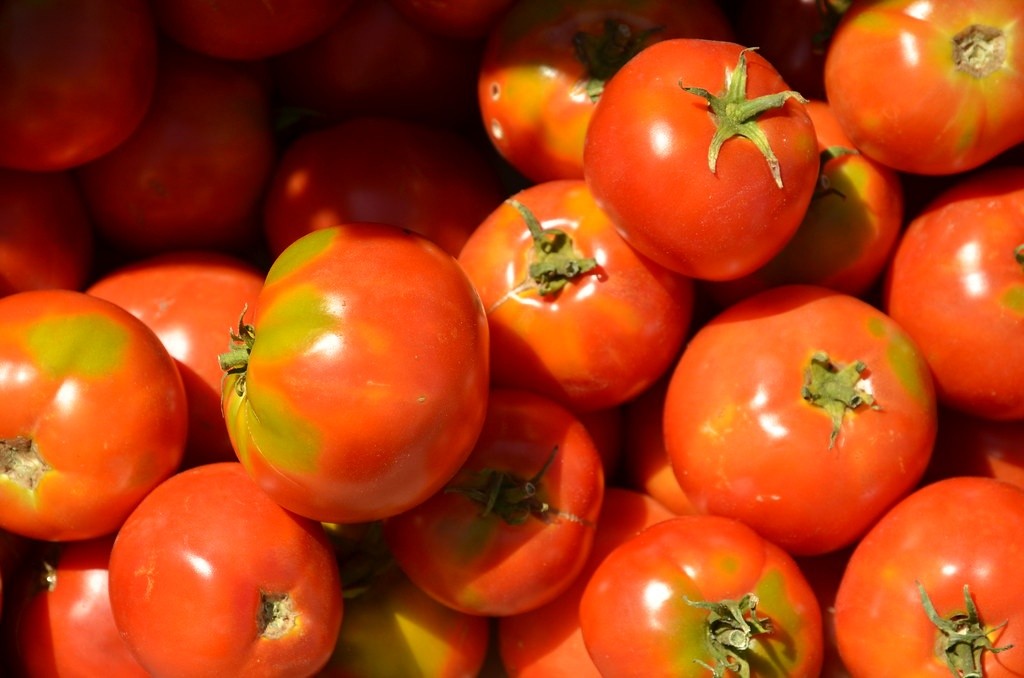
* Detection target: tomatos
[0,0,1024,678]
